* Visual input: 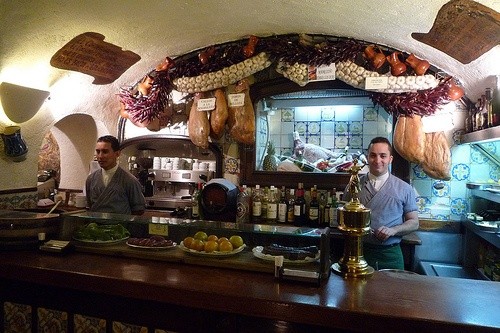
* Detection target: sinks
[472,221,500,228]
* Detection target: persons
[343,137,419,273]
[85,135,147,216]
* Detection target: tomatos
[183,231,243,252]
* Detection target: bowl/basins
[153,157,216,172]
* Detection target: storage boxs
[467,212,484,221]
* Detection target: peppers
[167,41,262,78]
[261,35,365,66]
[371,76,453,118]
[74,223,130,241]
[116,68,171,126]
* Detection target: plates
[125,241,177,249]
[178,241,247,258]
[72,234,129,245]
[484,188,500,194]
[253,246,320,264]
[474,220,500,228]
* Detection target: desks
[401,232,423,274]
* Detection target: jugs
[137,35,260,96]
[362,44,386,69]
[387,51,407,77]
[439,76,464,102]
[405,52,429,77]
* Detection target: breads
[262,243,318,260]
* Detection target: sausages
[127,237,173,246]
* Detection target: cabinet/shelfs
[463,183,500,282]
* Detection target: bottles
[236,184,250,223]
[464,87,500,133]
[252,182,348,231]
[191,181,206,219]
[357,151,367,164]
[291,131,305,157]
[293,160,322,172]
[334,146,350,163]
[479,242,500,281]
[327,161,355,173]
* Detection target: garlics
[331,59,440,93]
[173,52,272,94]
[276,57,309,81]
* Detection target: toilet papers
[75,193,87,208]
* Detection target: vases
[242,35,259,58]
[386,51,407,77]
[441,74,466,103]
[363,44,386,69]
[137,75,155,97]
[197,47,215,64]
[155,56,176,73]
[404,53,431,77]
